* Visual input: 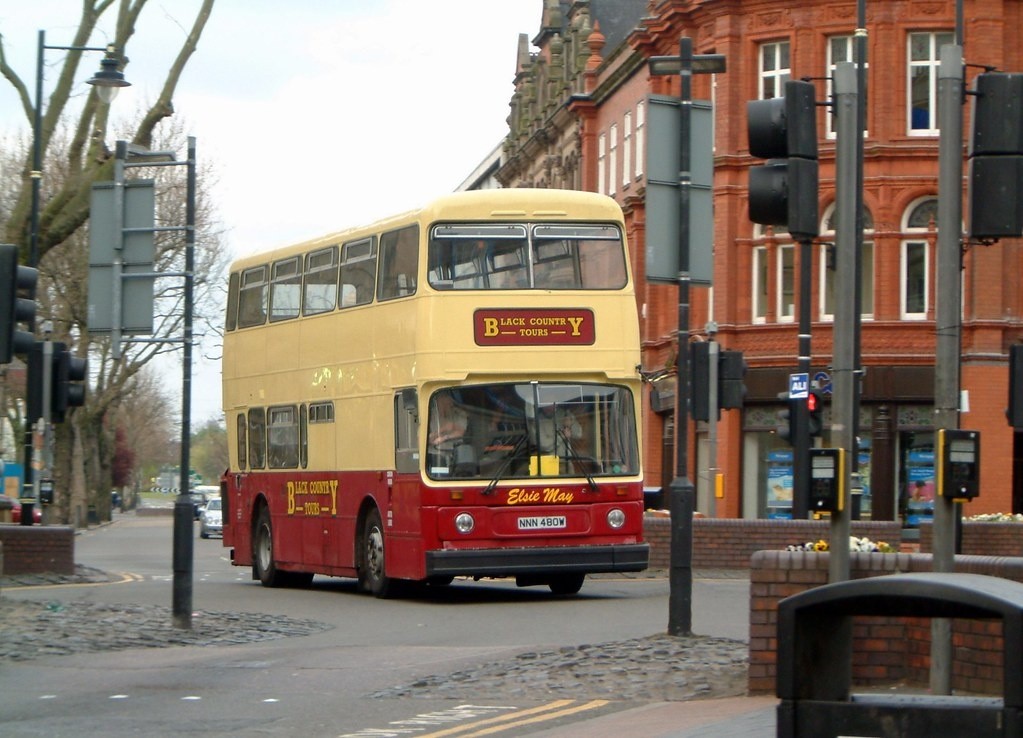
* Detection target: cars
[0,495,43,524]
[189,485,223,538]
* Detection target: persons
[110,487,120,510]
[211,502,220,509]
[774,484,794,501]
[910,481,931,502]
[426,390,583,477]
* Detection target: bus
[219,188,650,600]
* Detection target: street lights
[17,29,131,526]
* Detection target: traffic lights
[776,390,795,446]
[747,79,816,245]
[51,342,88,422]
[0,242,39,365]
[807,388,822,439]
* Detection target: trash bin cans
[775,571,1023,738]
[643,486,663,510]
